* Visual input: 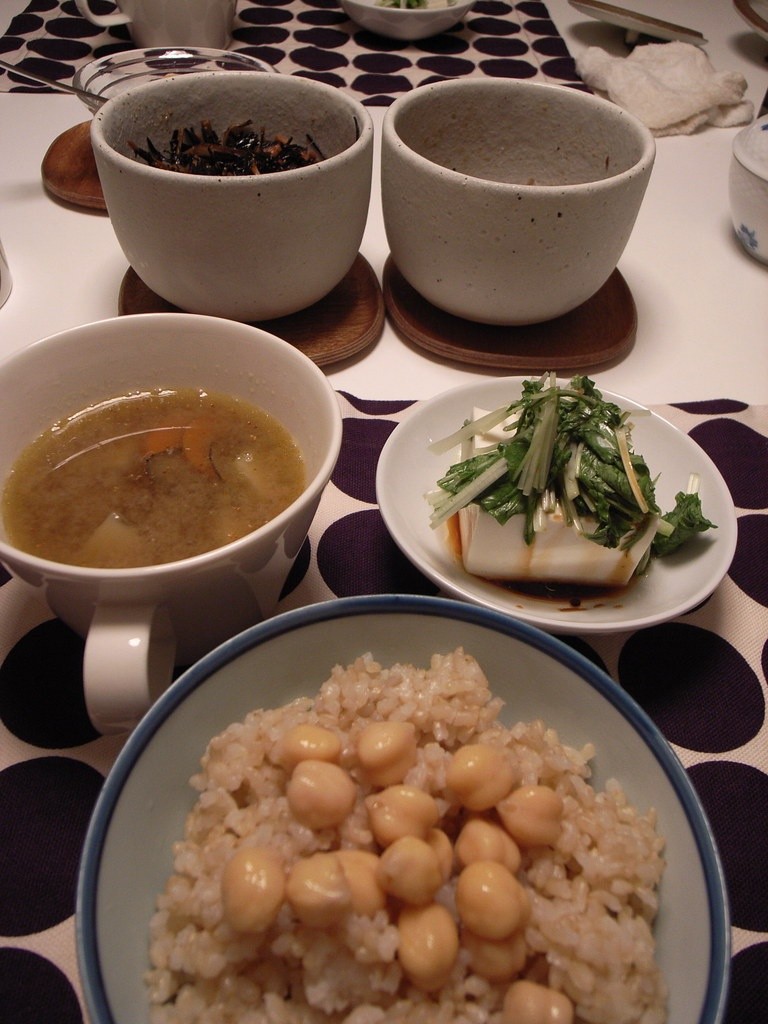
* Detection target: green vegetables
[427,369,718,573]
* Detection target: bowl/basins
[381,76,657,329]
[341,0,473,40]
[92,76,372,323]
[77,48,276,115]
[72,594,731,1024]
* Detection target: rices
[141,646,675,1024]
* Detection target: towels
[569,40,755,140]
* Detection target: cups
[81,0,236,49]
[1,315,341,740]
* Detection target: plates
[380,251,638,372]
[42,120,105,214]
[375,379,736,634]
[117,253,382,367]
[732,0,768,40]
